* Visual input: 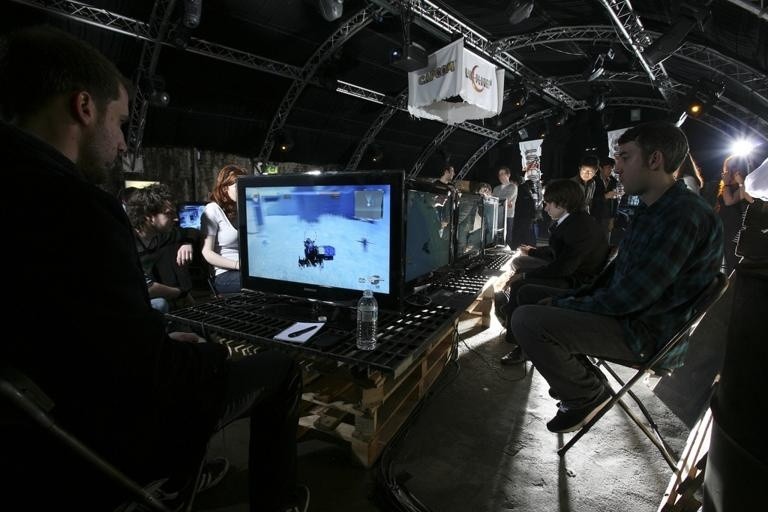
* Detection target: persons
[198,164,248,292]
[1,20,311,512]
[121,182,196,314]
[432,162,455,187]
[476,120,767,433]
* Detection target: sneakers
[165,455,231,502]
[280,480,313,512]
[500,344,527,365]
[548,376,615,434]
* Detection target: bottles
[357,290,378,350]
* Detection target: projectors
[387,46,428,73]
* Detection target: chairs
[554,268,730,471]
[0,364,251,511]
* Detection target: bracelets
[175,287,189,301]
[234,261,237,269]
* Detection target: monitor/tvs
[405,178,456,300]
[455,189,508,266]
[176,201,211,233]
[237,169,405,309]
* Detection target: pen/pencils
[288,326,317,338]
